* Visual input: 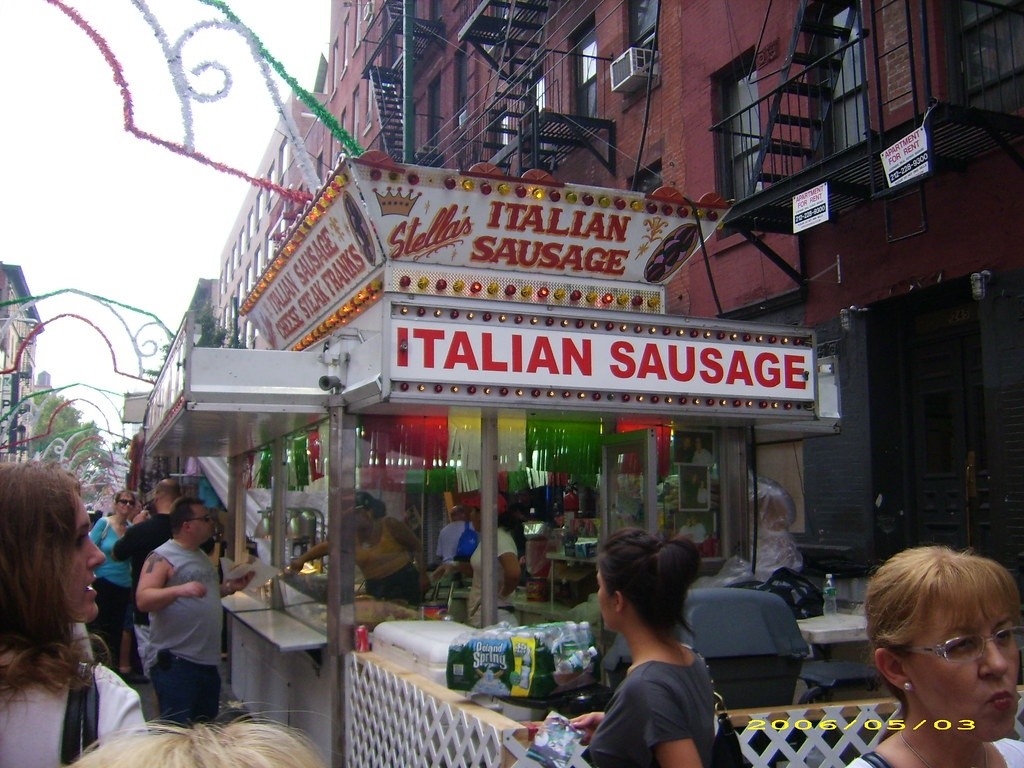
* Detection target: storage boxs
[564,544,576,557]
[568,519,600,537]
[471,683,614,722]
[600,427,728,573]
[575,537,599,558]
[370,619,478,686]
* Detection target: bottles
[823,573,837,617]
[478,620,599,680]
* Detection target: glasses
[890,626,1024,664]
[118,499,136,506]
[190,514,210,523]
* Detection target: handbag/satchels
[682,642,745,768]
[454,519,478,562]
[721,567,827,662]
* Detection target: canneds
[355,625,369,654]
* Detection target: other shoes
[119,665,148,685]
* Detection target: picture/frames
[677,462,711,513]
[671,426,717,470]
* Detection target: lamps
[840,304,870,332]
[969,269,994,302]
[318,373,345,396]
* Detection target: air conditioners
[364,2,372,21]
[609,47,658,94]
[458,110,469,129]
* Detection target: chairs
[796,658,882,704]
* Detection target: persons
[284,491,521,629]
[840,544,1024,768]
[681,472,708,509]
[567,526,718,768]
[676,435,713,464]
[0,460,325,768]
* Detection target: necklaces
[900,724,989,768]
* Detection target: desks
[797,612,871,656]
